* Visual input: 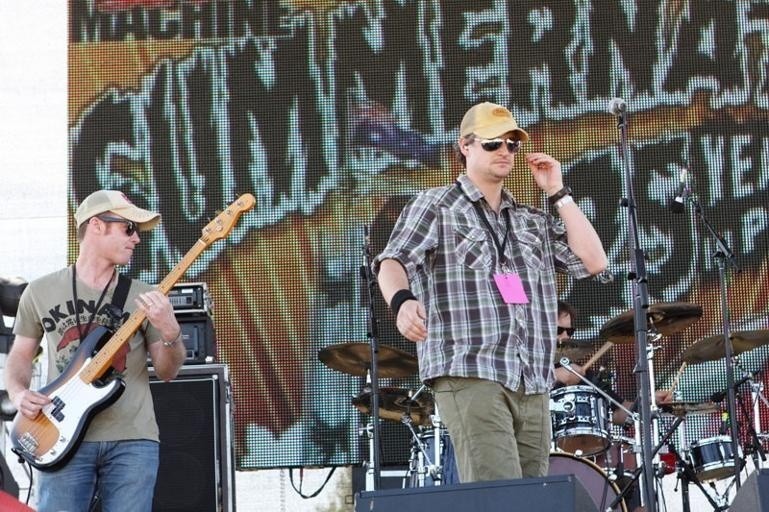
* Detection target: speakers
[151,363,235,512]
[354,473,597,511]
[727,469,769,512]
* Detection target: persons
[4,188,187,511]
[555,301,674,511]
[370,101,609,486]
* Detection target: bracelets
[548,187,573,203]
[162,328,183,347]
[553,195,573,210]
[390,288,417,316]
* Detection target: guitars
[9,192,256,473]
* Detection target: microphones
[608,96,627,115]
[671,160,688,213]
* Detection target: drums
[547,383,610,459]
[607,469,644,512]
[541,448,626,511]
[690,438,745,484]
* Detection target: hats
[74,190,160,232]
[460,102,529,143]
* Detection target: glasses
[557,327,574,337]
[474,137,521,154]
[88,215,137,236]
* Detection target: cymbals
[682,329,769,366]
[318,340,419,379]
[353,385,434,426]
[599,301,700,343]
[631,397,723,417]
